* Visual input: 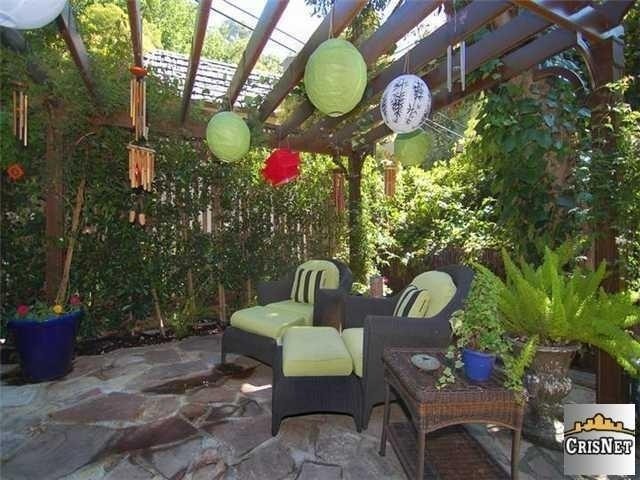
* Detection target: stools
[273,326,362,437]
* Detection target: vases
[7,310,84,380]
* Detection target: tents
[261,147,304,189]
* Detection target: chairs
[222,259,353,366]
[339,263,471,430]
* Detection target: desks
[378,346,525,480]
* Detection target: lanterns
[1,0,74,33]
[206,112,252,165]
[304,38,369,117]
[378,74,436,136]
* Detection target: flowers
[0,290,81,319]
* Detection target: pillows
[298,259,340,289]
[393,281,432,318]
[290,267,327,304]
[410,270,457,317]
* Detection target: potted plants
[468,237,640,435]
[434,273,523,392]
